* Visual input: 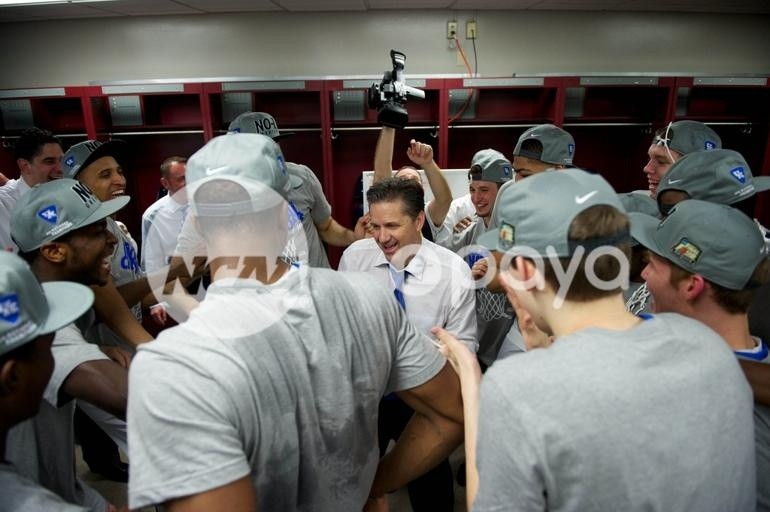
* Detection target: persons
[356,98,453,245]
[1,106,385,511]
[331,179,474,512]
[127,128,464,512]
[433,113,769,512]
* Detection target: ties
[388,264,407,311]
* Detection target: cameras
[368,50,426,130]
